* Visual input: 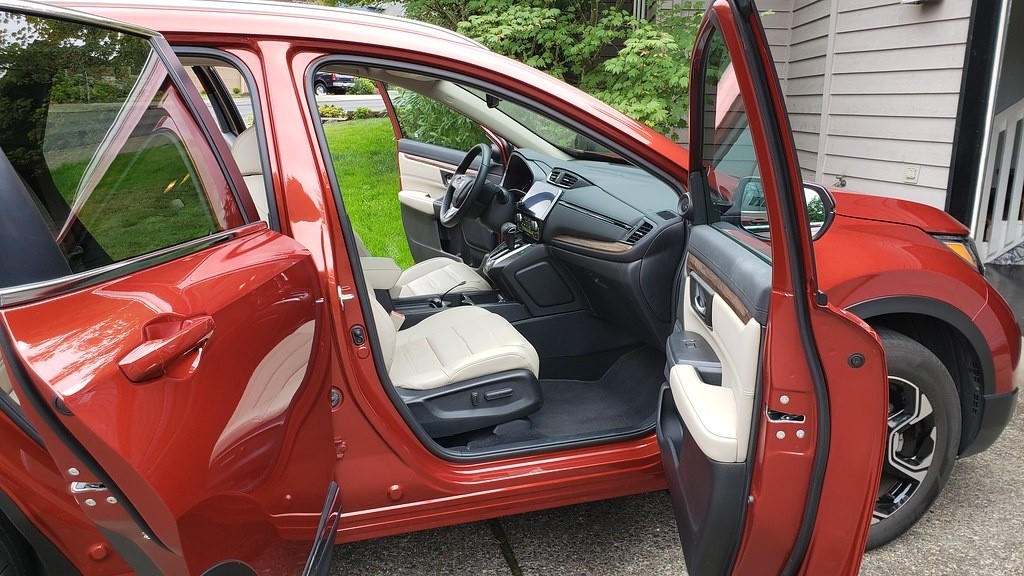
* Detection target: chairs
[228,125,544,440]
[251,121,491,301]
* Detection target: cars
[0,0,1023,576]
[313,72,355,96]
